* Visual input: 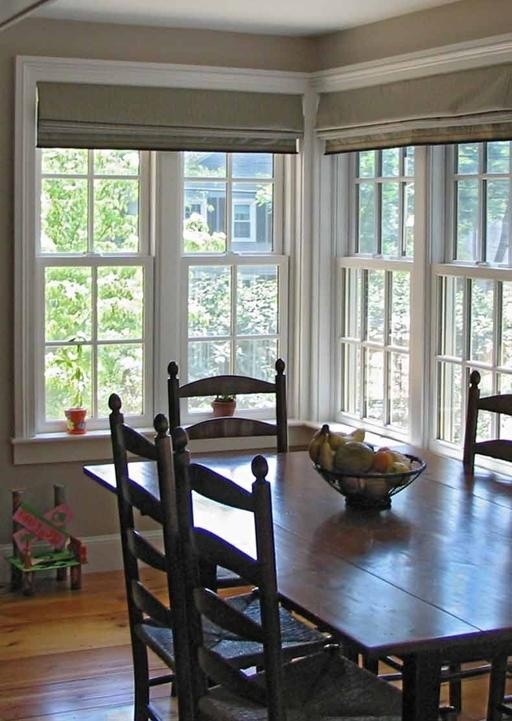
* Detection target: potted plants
[52,342,87,433]
[210,388,237,417]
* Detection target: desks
[83,444,512,721]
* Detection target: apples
[386,462,409,473]
[375,451,393,472]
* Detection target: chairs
[486,655,512,721]
[167,358,293,686]
[344,369,512,719]
[108,393,332,721]
[170,426,458,721]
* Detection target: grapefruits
[336,441,374,473]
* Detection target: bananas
[309,425,364,471]
